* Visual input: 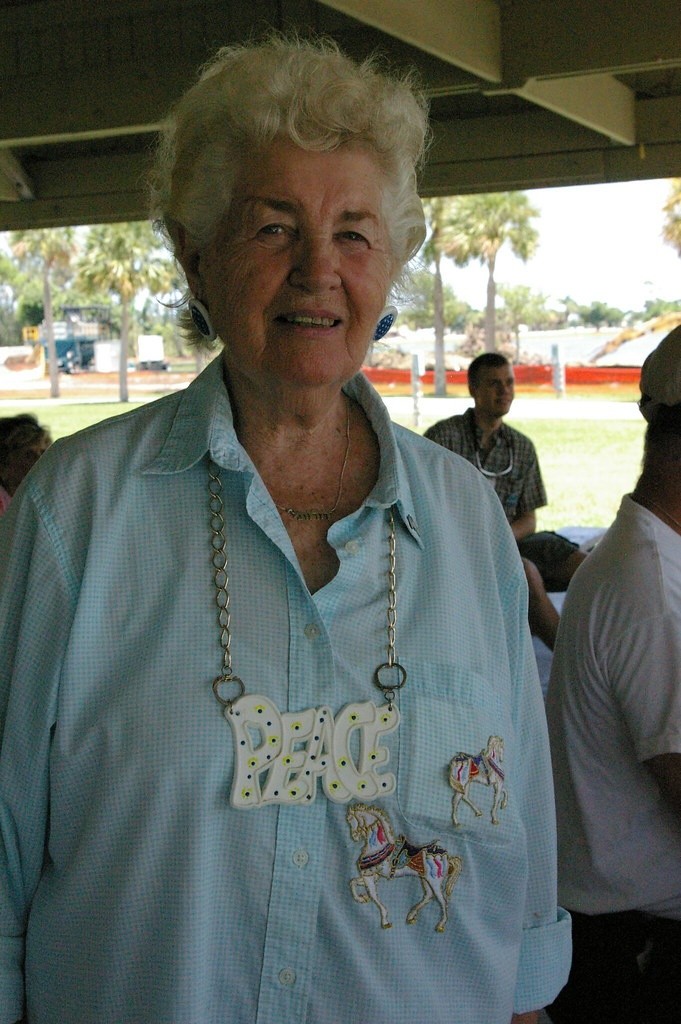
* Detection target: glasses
[475,440,514,480]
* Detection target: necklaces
[202,452,406,810]
[274,392,351,521]
[474,440,513,478]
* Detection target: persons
[0,34,574,1024]
[0,412,53,512]
[546,327,681,1024]
[423,353,590,654]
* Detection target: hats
[639,324,681,424]
